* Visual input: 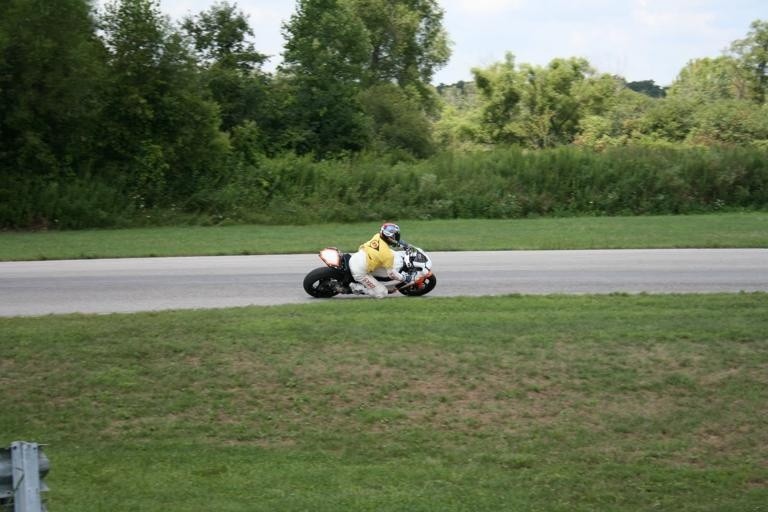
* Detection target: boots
[350,283,364,295]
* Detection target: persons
[348,223,412,298]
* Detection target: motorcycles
[302,239,438,298]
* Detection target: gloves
[406,276,412,282]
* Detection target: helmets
[380,223,400,247]
